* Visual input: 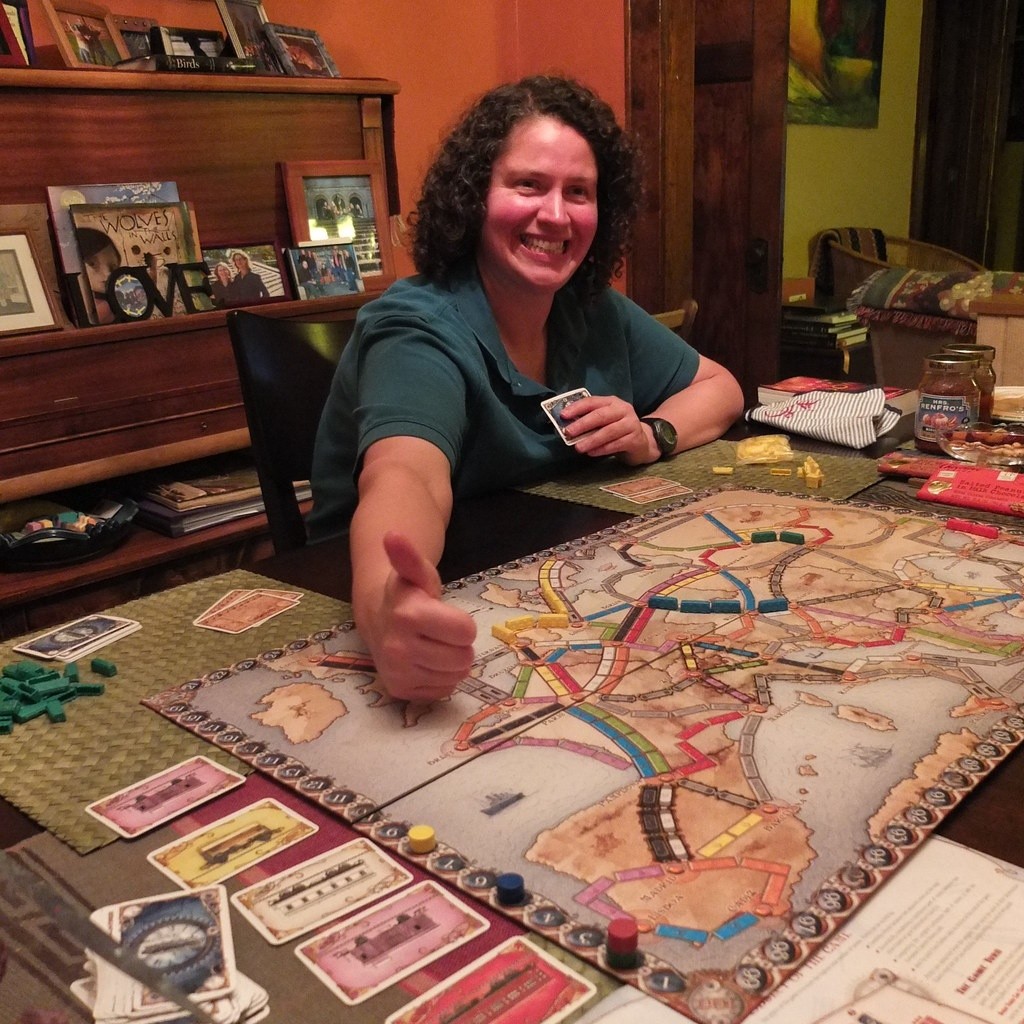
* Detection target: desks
[0,400,1024,1023]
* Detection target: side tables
[780,343,875,384]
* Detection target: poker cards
[12,609,143,665]
[59,749,596,1024]
[541,381,596,448]
[193,584,303,638]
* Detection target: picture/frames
[199,237,293,309]
[0,0,38,69]
[42,0,132,71]
[215,0,285,75]
[263,23,340,78]
[1,226,65,337]
[280,160,398,292]
[112,14,157,60]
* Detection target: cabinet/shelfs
[1,65,399,640]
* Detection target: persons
[309,70,746,704]
[202,250,271,305]
[299,250,357,298]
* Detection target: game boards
[136,484,1024,1024]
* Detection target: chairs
[225,311,357,556]
[826,234,991,299]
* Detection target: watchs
[638,416,679,462]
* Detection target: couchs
[850,265,1024,392]
